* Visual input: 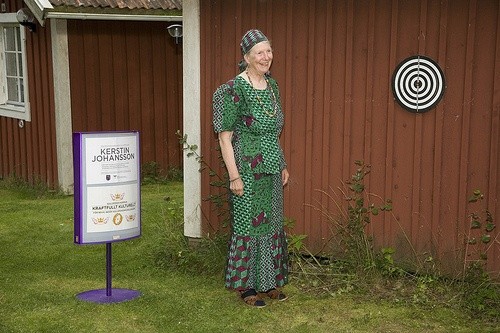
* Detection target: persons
[212,30,289,309]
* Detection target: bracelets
[229,175,242,183]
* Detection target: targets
[391,55,446,114]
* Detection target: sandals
[259,289,288,301]
[239,289,267,308]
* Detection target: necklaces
[246,71,278,118]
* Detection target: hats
[242,30,268,55]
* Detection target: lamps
[167,24,183,44]
[16,7,37,34]
